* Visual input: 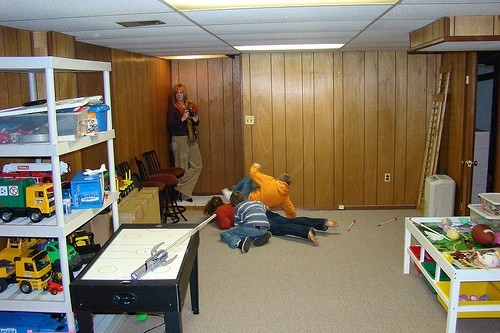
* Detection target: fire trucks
[2,159,71,191]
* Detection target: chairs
[117,150,189,223]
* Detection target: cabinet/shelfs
[0,56,119,333]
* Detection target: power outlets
[246,116,254,124]
[385,173,390,182]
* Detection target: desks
[69,223,199,333]
[404,217,500,333]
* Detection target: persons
[167,83,203,202]
[203,196,339,244]
[219,191,272,254]
[221,162,297,218]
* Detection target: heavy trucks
[105,170,134,212]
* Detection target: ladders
[418,71,451,212]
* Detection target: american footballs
[472,224,495,245]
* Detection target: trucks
[0,229,102,295]
[0,176,56,223]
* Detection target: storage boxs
[467,193,500,232]
[0,310,79,333]
[0,104,110,144]
[70,172,105,209]
[82,186,161,247]
